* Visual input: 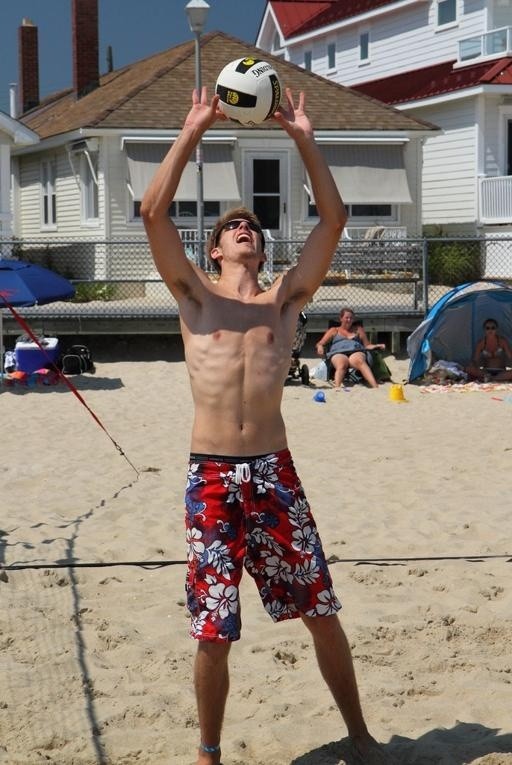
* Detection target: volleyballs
[215,57,284,128]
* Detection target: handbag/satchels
[62,345,92,375]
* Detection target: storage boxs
[15,338,59,376]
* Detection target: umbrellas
[0,258,76,384]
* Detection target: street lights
[184,2,209,273]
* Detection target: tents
[404,279,512,387]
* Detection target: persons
[135,86,397,765]
[315,307,386,389]
[465,318,512,381]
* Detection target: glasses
[215,220,262,248]
[486,326,495,330]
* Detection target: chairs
[326,320,368,385]
[363,227,384,246]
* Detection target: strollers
[288,311,309,384]
